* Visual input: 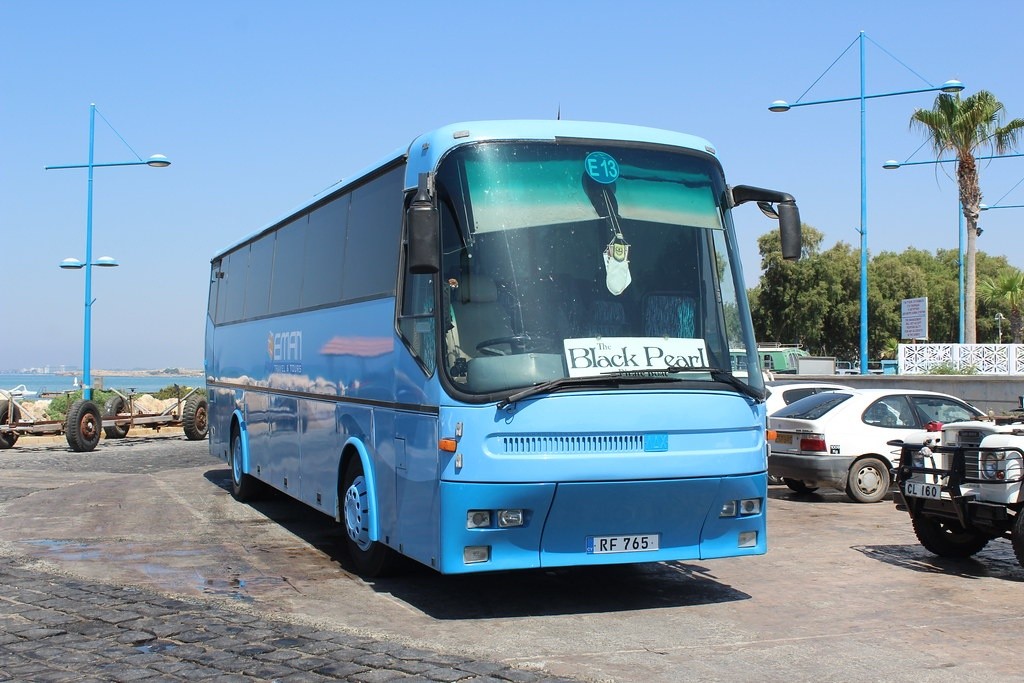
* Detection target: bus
[204,108,803,574]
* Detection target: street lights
[882,92,1024,343]
[44,103,171,401]
[769,30,965,375]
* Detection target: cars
[763,380,855,417]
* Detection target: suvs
[766,389,995,503]
[887,393,1024,571]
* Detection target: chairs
[900,406,926,427]
[529,304,572,356]
[587,302,634,339]
[872,404,896,428]
[641,289,698,340]
[453,276,517,359]
